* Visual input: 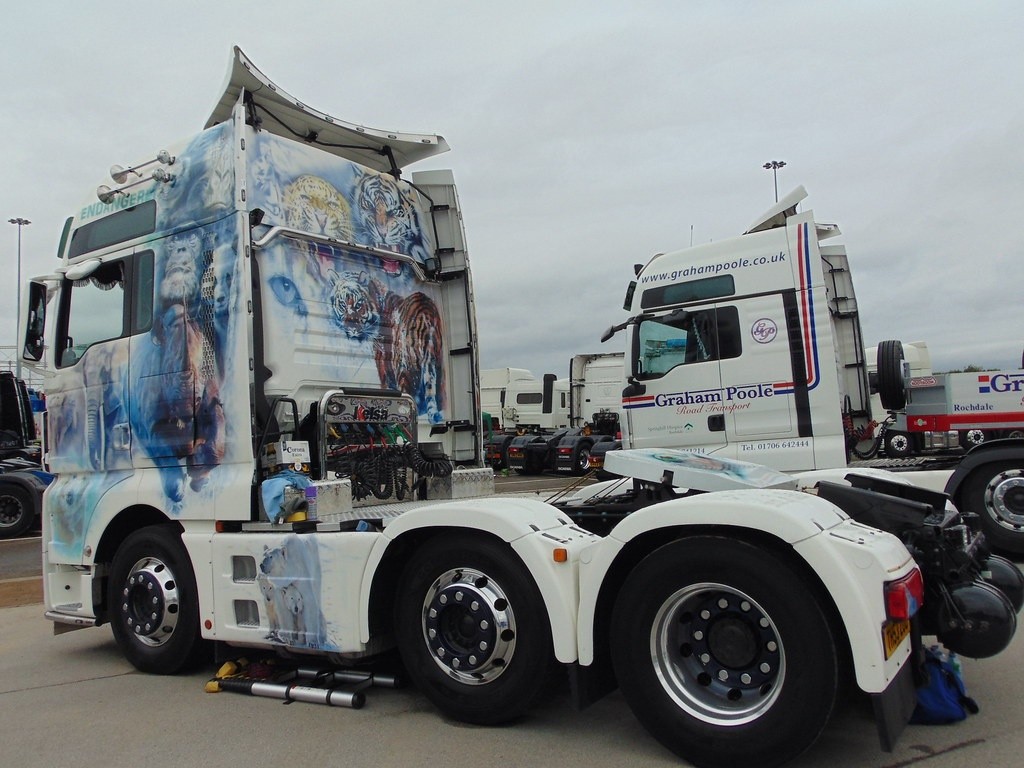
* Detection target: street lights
[761,160,789,204]
[8,216,32,348]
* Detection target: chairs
[696,317,714,363]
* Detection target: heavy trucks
[15,43,1024,767]
[475,185,1024,562]
[0,368,56,540]
